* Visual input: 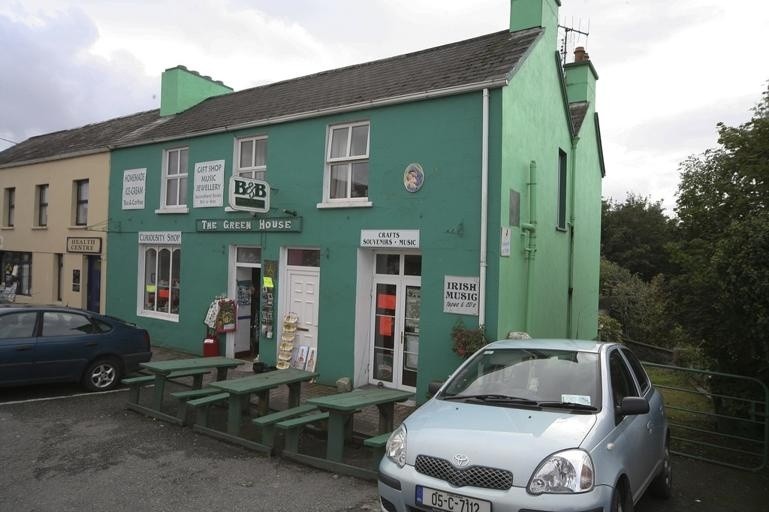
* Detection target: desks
[138,355,416,462]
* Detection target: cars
[0,300,152,393]
[375,337,672,512]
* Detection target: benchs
[121,369,394,482]
